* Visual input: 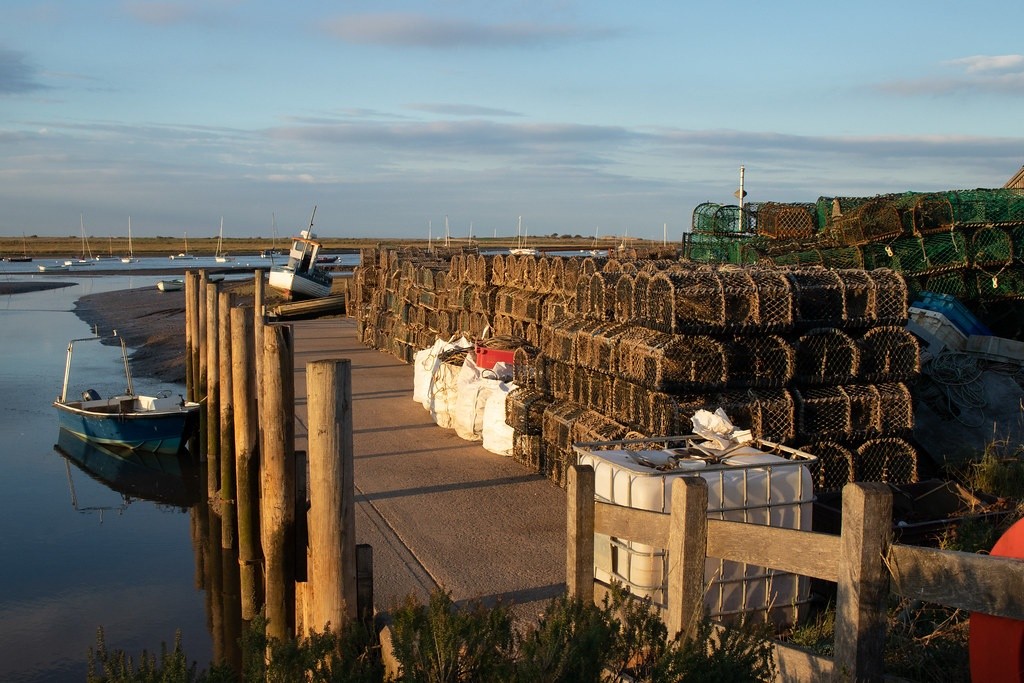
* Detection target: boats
[268,203,334,299]
[52,334,201,444]
[53,425,202,514]
[39,265,68,272]
[157,273,226,291]
[315,255,338,263]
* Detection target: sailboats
[75,213,101,264]
[587,227,634,256]
[508,216,540,257]
[214,217,236,264]
[7,231,37,262]
[95,233,121,262]
[260,213,282,258]
[170,232,195,260]
[120,214,140,264]
